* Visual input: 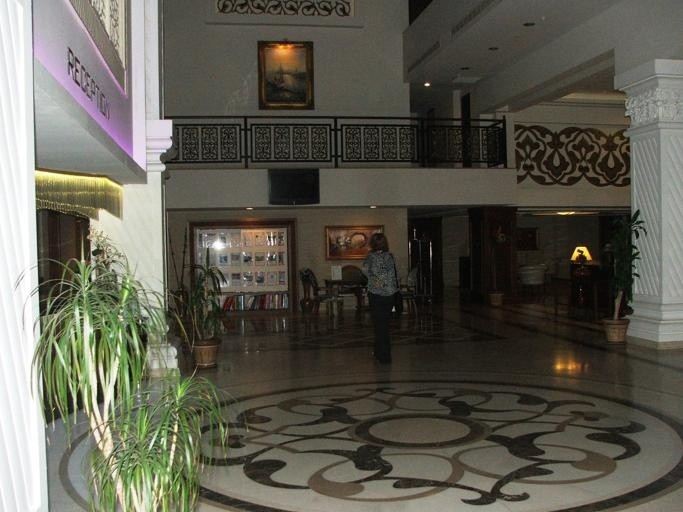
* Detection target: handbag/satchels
[394,293,404,314]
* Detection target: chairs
[396,266,419,320]
[299,268,337,319]
[342,266,368,307]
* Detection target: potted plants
[595,209,647,344]
[177,247,232,369]
[478,222,511,308]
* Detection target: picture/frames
[326,224,385,260]
[257,40,314,110]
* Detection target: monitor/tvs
[269,168,319,205]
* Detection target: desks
[325,279,364,318]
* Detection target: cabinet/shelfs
[188,220,295,320]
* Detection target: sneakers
[374,352,393,364]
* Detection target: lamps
[571,245,593,321]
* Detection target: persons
[361,233,401,364]
